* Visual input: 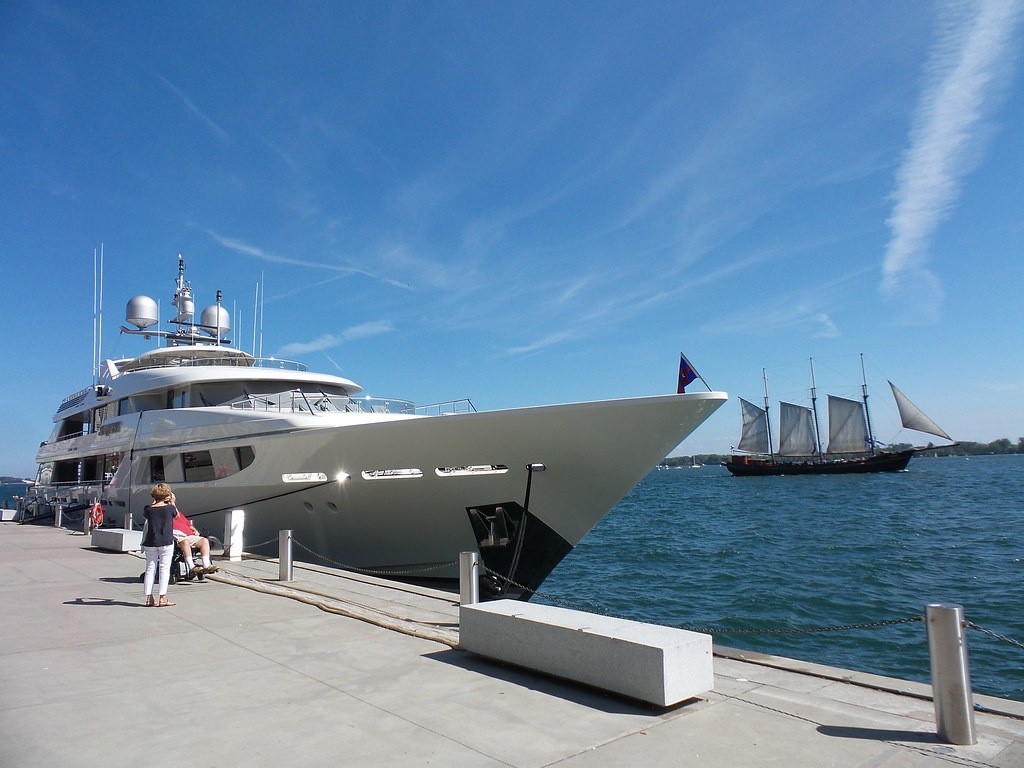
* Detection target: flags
[677,354,699,394]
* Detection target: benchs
[460,598,715,709]
[91,528,143,554]
[0,508,19,521]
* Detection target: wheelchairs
[168,527,219,586]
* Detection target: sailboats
[720,351,961,477]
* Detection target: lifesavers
[90,504,103,524]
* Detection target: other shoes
[146,599,157,607]
[160,599,176,606]
[190,564,220,576]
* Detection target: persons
[140,483,179,606]
[776,458,845,466]
[165,493,219,574]
[25,486,37,516]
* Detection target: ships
[20,242,731,605]
